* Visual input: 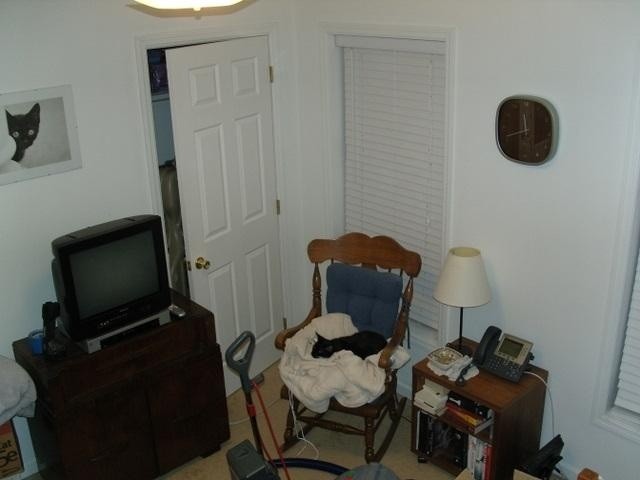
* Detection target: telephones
[473,325,533,384]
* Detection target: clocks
[495,94,561,166]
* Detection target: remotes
[167,303,187,319]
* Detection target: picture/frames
[0,84,83,185]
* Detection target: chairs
[275,232,423,464]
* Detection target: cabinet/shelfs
[12,289,230,480]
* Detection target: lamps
[434,247,491,357]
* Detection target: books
[413,377,495,480]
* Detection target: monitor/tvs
[515,433,563,480]
[50,214,171,341]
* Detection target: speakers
[41,302,60,340]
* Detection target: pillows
[325,263,403,341]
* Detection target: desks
[411,336,549,480]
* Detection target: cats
[5,103,40,162]
[311,330,387,360]
